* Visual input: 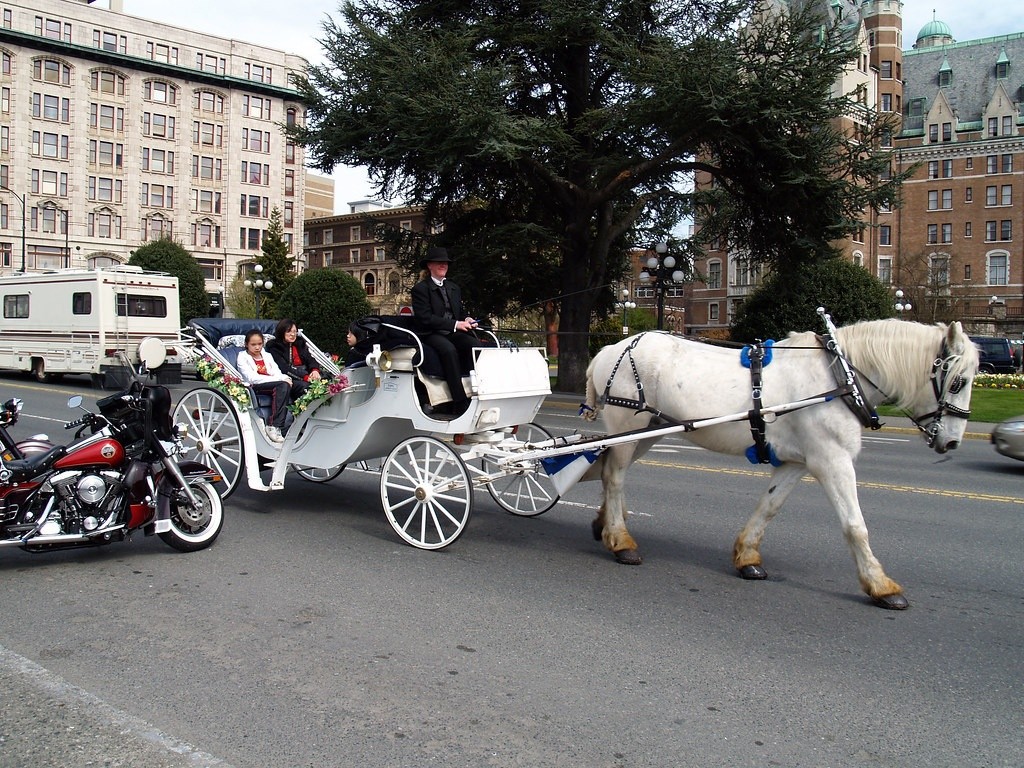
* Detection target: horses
[578,317,979,609]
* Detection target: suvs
[967,336,1023,377]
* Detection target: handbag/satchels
[290,365,308,375]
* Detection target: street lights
[614,289,636,339]
[218,286,224,318]
[244,265,273,319]
[639,243,684,333]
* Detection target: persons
[326,320,377,380]
[266,320,332,403]
[410,246,487,415]
[237,329,310,442]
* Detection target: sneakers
[265,425,285,443]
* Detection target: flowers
[191,353,249,409]
[288,372,350,415]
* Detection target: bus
[0,264,182,384]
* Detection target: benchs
[191,319,334,431]
[301,360,373,438]
[377,315,479,375]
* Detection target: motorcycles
[0,352,224,553]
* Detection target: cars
[990,414,1024,464]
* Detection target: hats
[423,248,452,261]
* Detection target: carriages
[172,306,981,611]
